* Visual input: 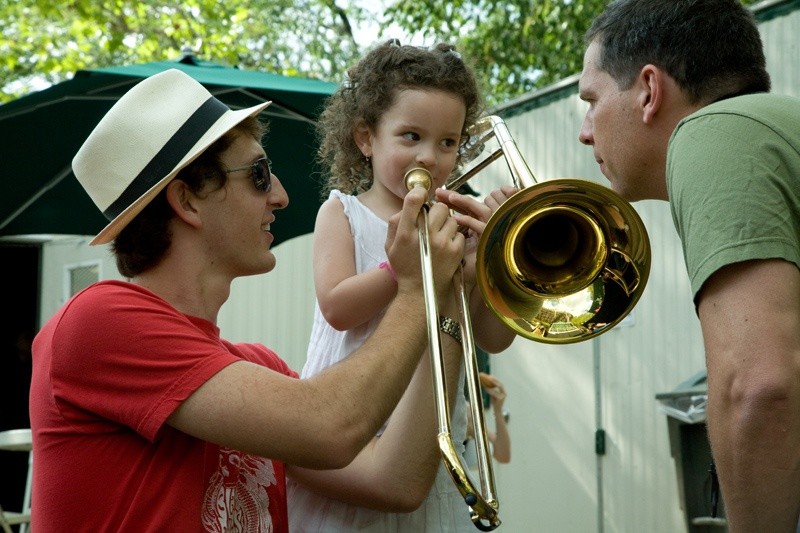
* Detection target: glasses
[186,158,273,191]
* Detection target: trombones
[403,112,655,533]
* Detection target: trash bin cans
[656,390,729,533]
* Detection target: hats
[71,68,273,246]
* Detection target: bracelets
[439,316,462,343]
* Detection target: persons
[578,1,800,533]
[28,44,510,533]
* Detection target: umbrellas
[0,47,355,284]
